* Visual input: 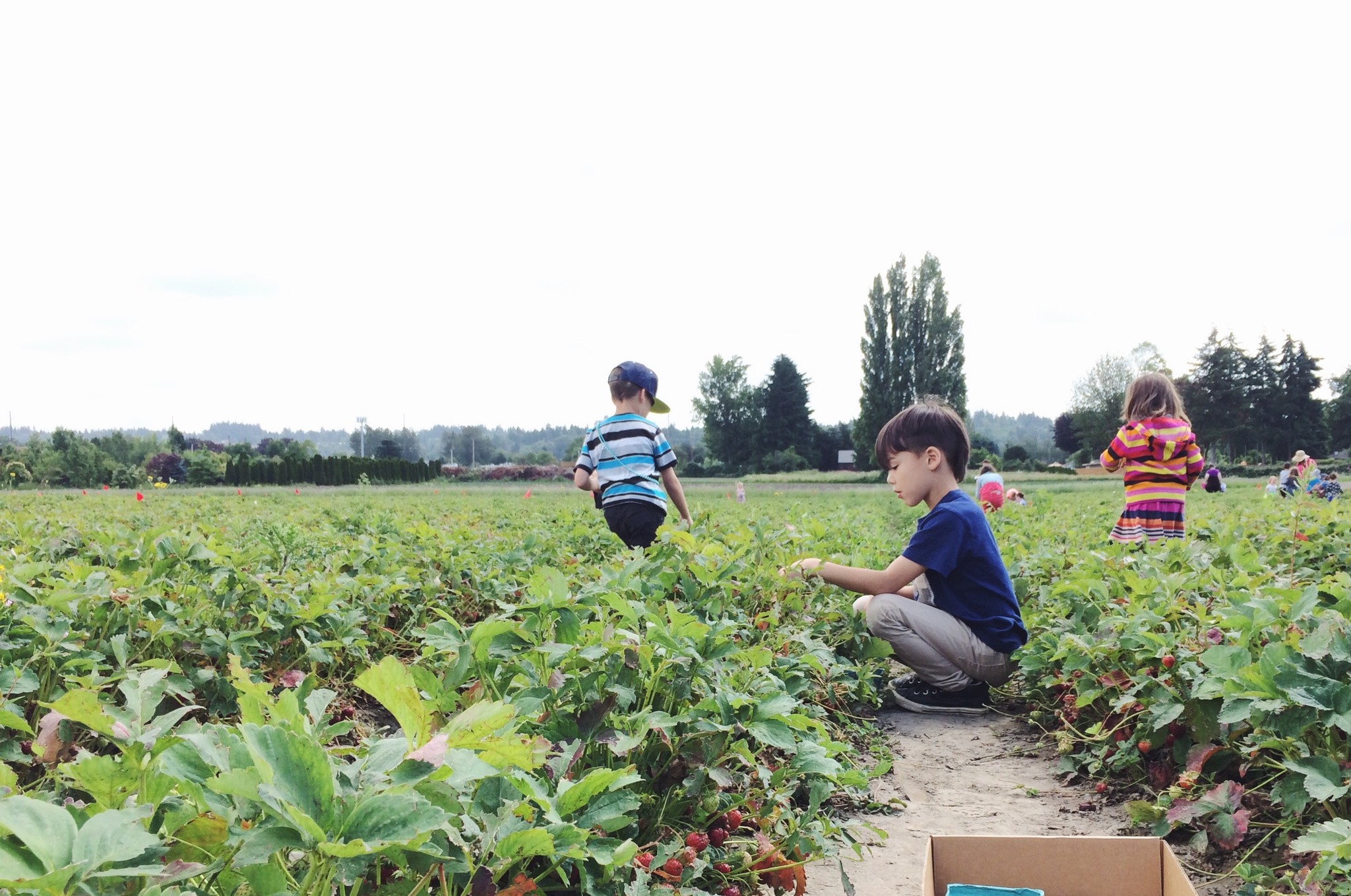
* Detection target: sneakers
[891,682,990,717]
[889,673,925,691]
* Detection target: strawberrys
[21,655,1351,896]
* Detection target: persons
[1201,464,1225,493]
[574,360,693,552]
[1262,450,1344,502]
[976,459,1026,514]
[734,481,746,504]
[1100,372,1203,553]
[778,394,1028,715]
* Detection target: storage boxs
[919,831,1204,896]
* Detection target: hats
[1292,450,1310,463]
[608,361,670,414]
